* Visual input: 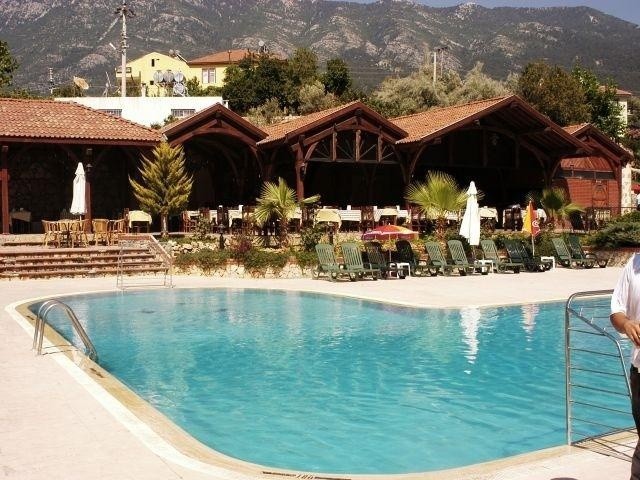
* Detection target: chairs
[8,205,152,248]
[180,204,548,233]
[311,232,610,280]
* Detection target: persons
[608,252,640,480]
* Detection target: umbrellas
[361,221,419,279]
[522,200,542,261]
[457,180,481,274]
[69,162,88,233]
